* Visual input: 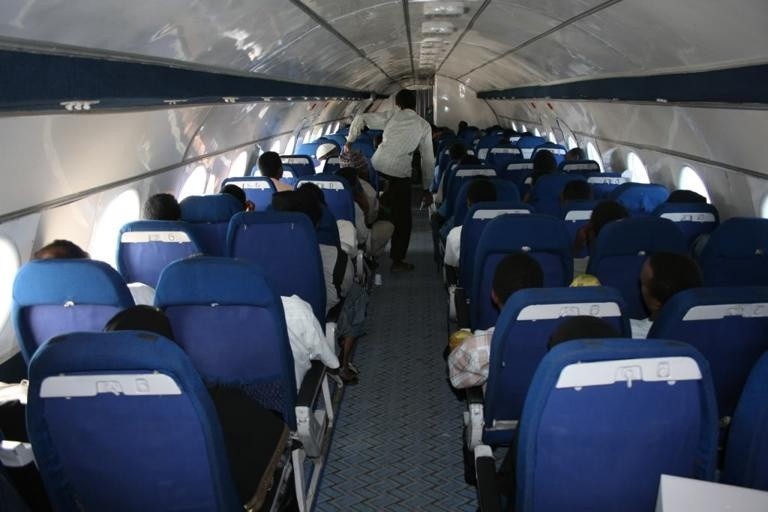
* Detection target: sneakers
[338,362,356,380]
[367,260,379,270]
[391,260,414,273]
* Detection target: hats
[316,143,336,160]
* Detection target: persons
[35,135,394,510]
[344,90,435,273]
[426,120,706,397]
